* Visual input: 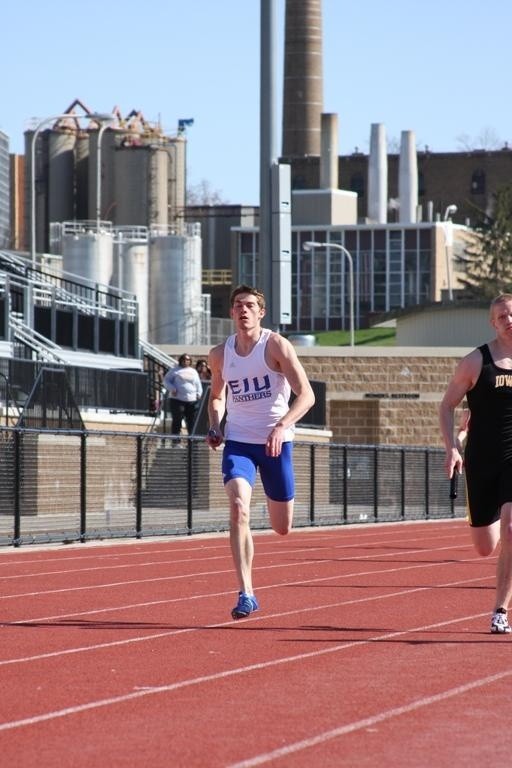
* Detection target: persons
[161,351,205,446]
[437,292,512,638]
[206,282,316,620]
[195,357,211,435]
[454,408,472,457]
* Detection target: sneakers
[489,607,512,634]
[230,591,261,620]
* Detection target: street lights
[301,238,357,350]
[442,202,458,301]
[23,110,114,362]
